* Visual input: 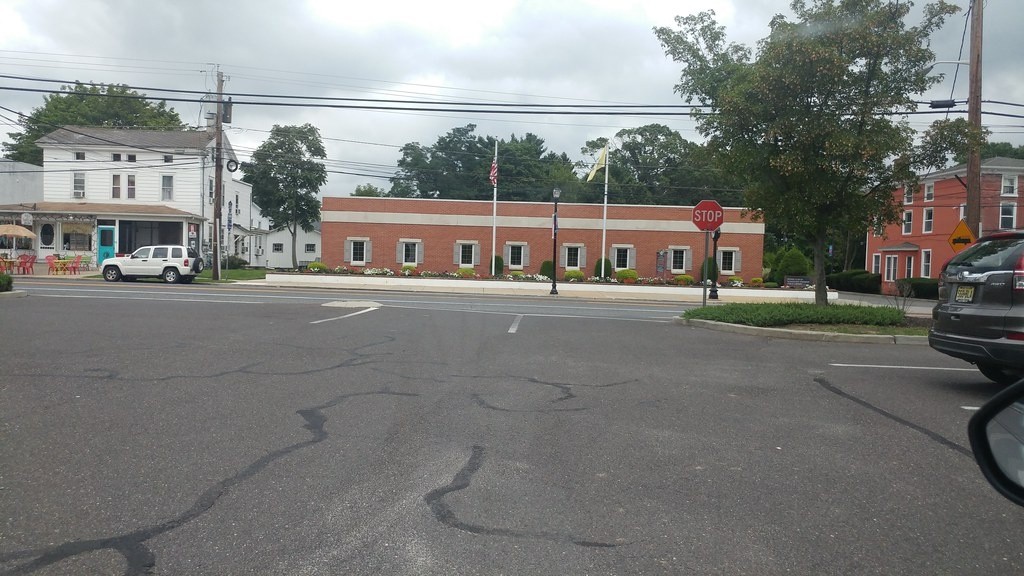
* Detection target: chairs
[0,253,94,276]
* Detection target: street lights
[200,149,221,280]
[551,187,562,294]
[920,60,982,241]
[709,226,719,299]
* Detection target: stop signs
[691,200,724,232]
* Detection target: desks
[0,259,23,275]
[52,260,73,275]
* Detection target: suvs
[927,228,1023,383]
[100,244,205,284]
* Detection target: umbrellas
[0,225,37,249]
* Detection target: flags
[586,149,605,182]
[489,156,497,187]
